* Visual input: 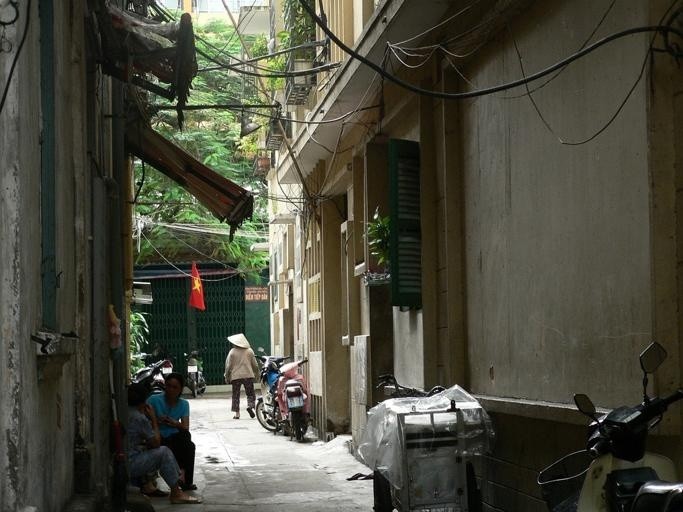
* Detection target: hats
[227,333,251,349]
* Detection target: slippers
[169,495,203,504]
[140,488,170,497]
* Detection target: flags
[188,262,205,310]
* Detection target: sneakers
[232,416,239,419]
[246,407,255,418]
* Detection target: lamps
[239,114,260,140]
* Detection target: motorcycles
[182,346,207,397]
[368,372,492,511]
[253,346,290,433]
[129,351,173,403]
[276,358,310,441]
[535,339,682,512]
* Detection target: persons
[141,372,197,497]
[222,333,261,419]
[127,383,204,505]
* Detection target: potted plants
[276,31,313,88]
